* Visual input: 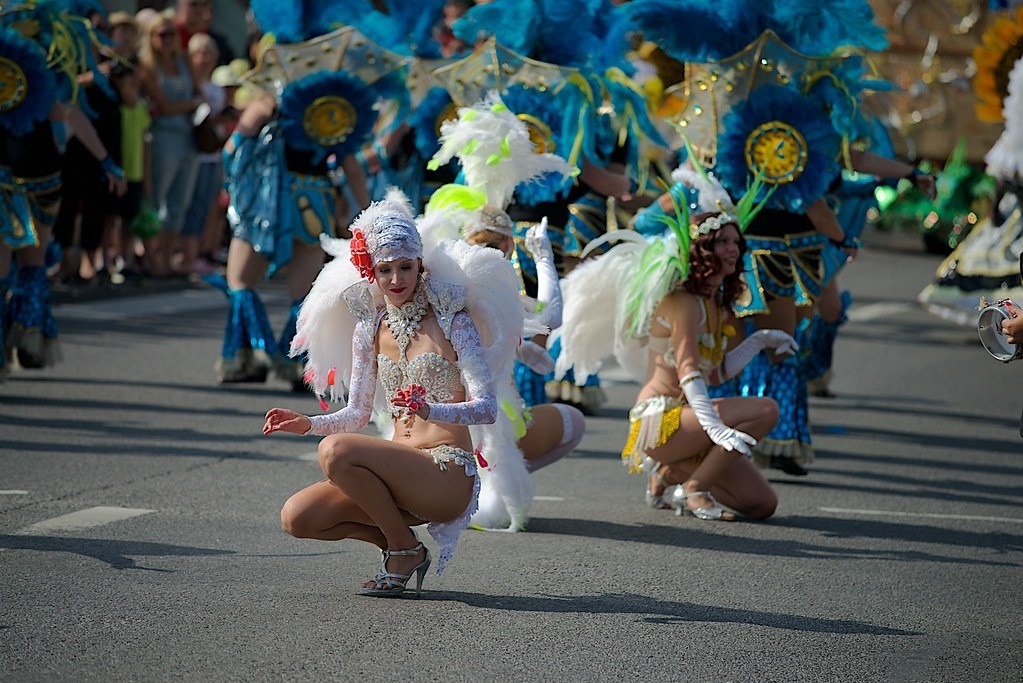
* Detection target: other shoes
[771,454,808,476]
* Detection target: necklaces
[381,288,427,371]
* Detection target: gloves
[717,329,800,385]
[680,371,757,458]
[524,216,563,329]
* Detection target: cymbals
[976,297,1023,364]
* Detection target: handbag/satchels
[190,110,219,155]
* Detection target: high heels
[672,483,736,522]
[638,455,670,510]
[224,365,270,384]
[356,527,432,598]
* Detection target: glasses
[155,32,176,40]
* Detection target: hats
[211,66,240,90]
[107,12,139,38]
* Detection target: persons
[620,210,800,522]
[1,0,1023,597]
